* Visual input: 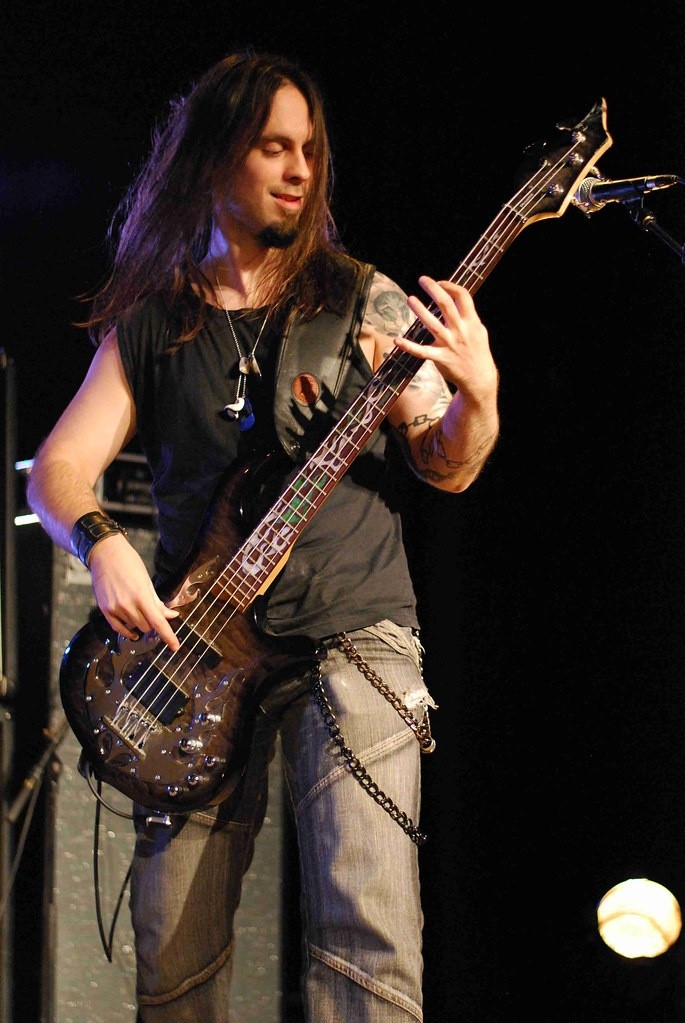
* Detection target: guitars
[57,92,616,816]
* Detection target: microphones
[571,172,680,213]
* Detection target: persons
[27,48,501,1023]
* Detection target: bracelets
[70,511,129,571]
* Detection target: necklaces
[210,248,272,432]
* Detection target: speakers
[11,523,296,1023]
